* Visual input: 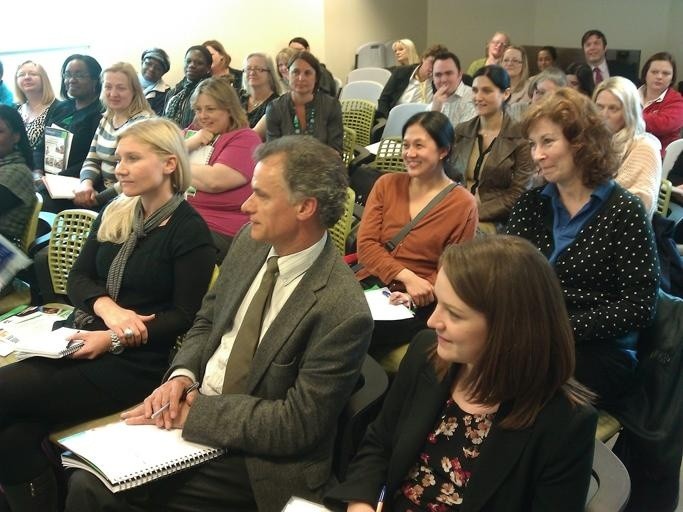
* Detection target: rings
[125,328,134,338]
[119,334,125,338]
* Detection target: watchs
[109,329,124,355]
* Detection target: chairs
[334,353,390,485]
[655,178,675,219]
[344,128,358,169]
[340,98,377,163]
[383,103,431,139]
[339,79,384,106]
[353,41,388,70]
[596,408,623,448]
[327,188,356,258]
[580,441,631,511]
[346,67,391,88]
[385,39,401,69]
[0,190,44,315]
[47,208,100,296]
[334,74,343,101]
[374,135,409,175]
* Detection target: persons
[288,38,336,97]
[178,77,265,261]
[369,45,449,145]
[424,52,479,137]
[468,31,511,79]
[239,52,277,141]
[62,137,373,512]
[321,235,598,512]
[505,88,659,407]
[202,40,243,88]
[73,61,158,208]
[276,48,296,94]
[501,46,534,109]
[0,60,14,107]
[162,45,214,128]
[0,120,218,511]
[591,76,663,223]
[528,46,559,76]
[634,52,683,162]
[355,111,480,352]
[0,105,37,303]
[580,30,640,99]
[136,47,172,116]
[265,52,345,159]
[11,60,63,154]
[382,39,421,74]
[32,54,106,212]
[443,65,536,241]
[567,61,594,99]
[505,67,567,123]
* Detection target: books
[47,386,234,493]
[43,126,68,174]
[42,173,100,200]
[51,122,74,171]
[15,327,90,357]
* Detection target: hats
[141,48,170,71]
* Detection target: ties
[593,67,605,83]
[222,255,279,398]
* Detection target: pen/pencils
[376,486,387,511]
[65,330,81,349]
[383,290,404,303]
[151,382,200,420]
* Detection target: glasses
[502,57,524,64]
[63,72,91,80]
[15,70,40,77]
[247,66,269,72]
[488,40,506,47]
